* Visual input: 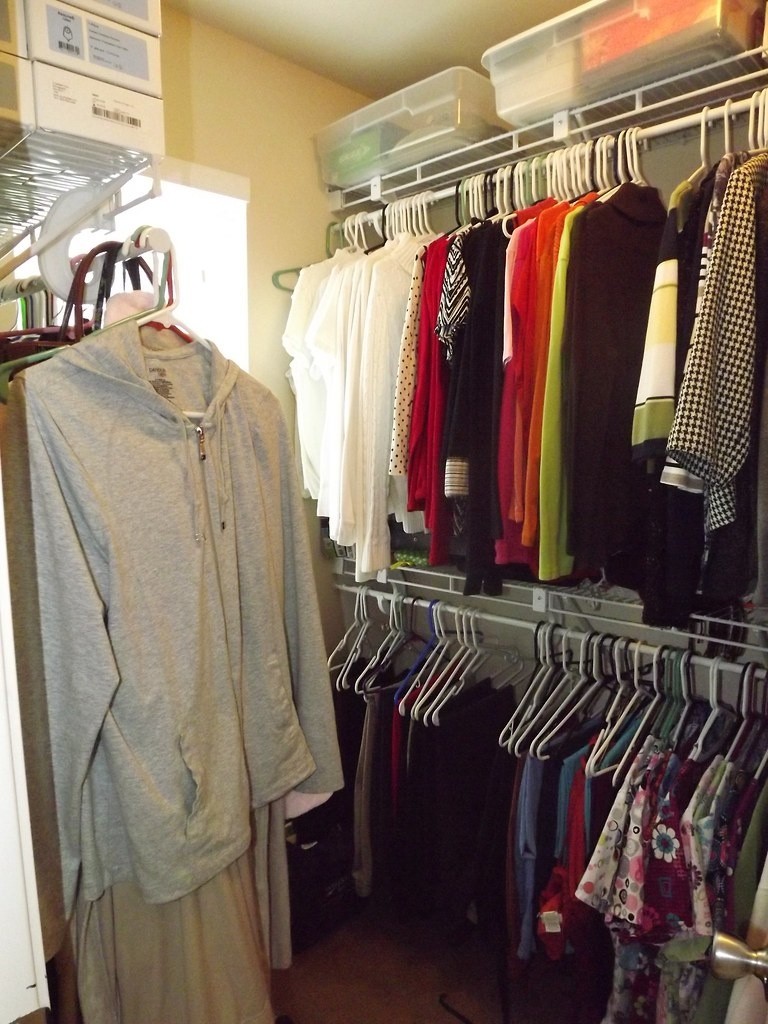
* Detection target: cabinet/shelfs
[329,88,768,684]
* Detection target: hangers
[0,226,213,407]
[327,585,768,788]
[271,89,767,294]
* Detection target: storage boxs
[307,0,768,200]
[0,0,166,238]
